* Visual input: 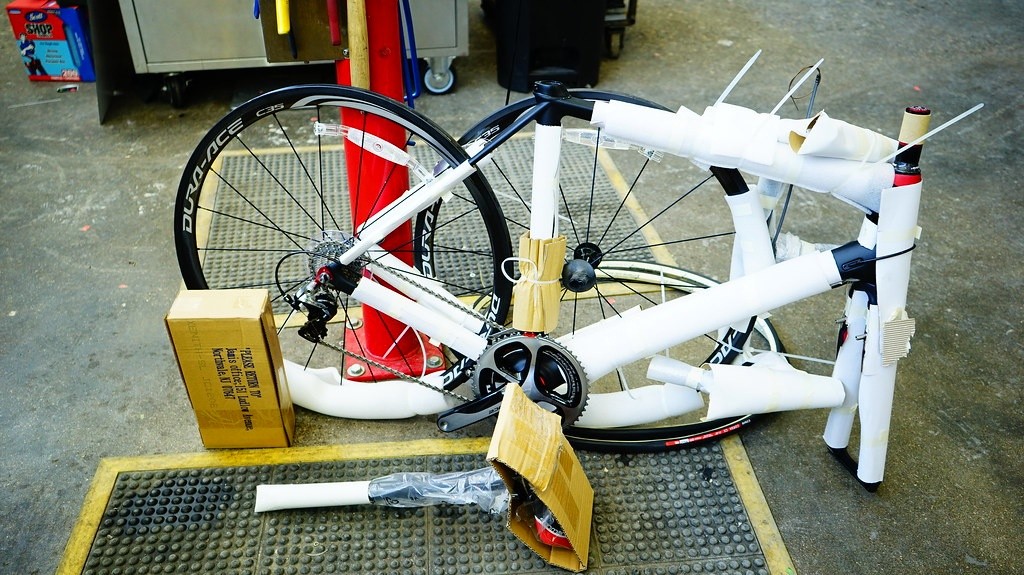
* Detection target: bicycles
[172,65,929,495]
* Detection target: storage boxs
[164,288,298,450]
[6,0,96,82]
[485,382,594,572]
